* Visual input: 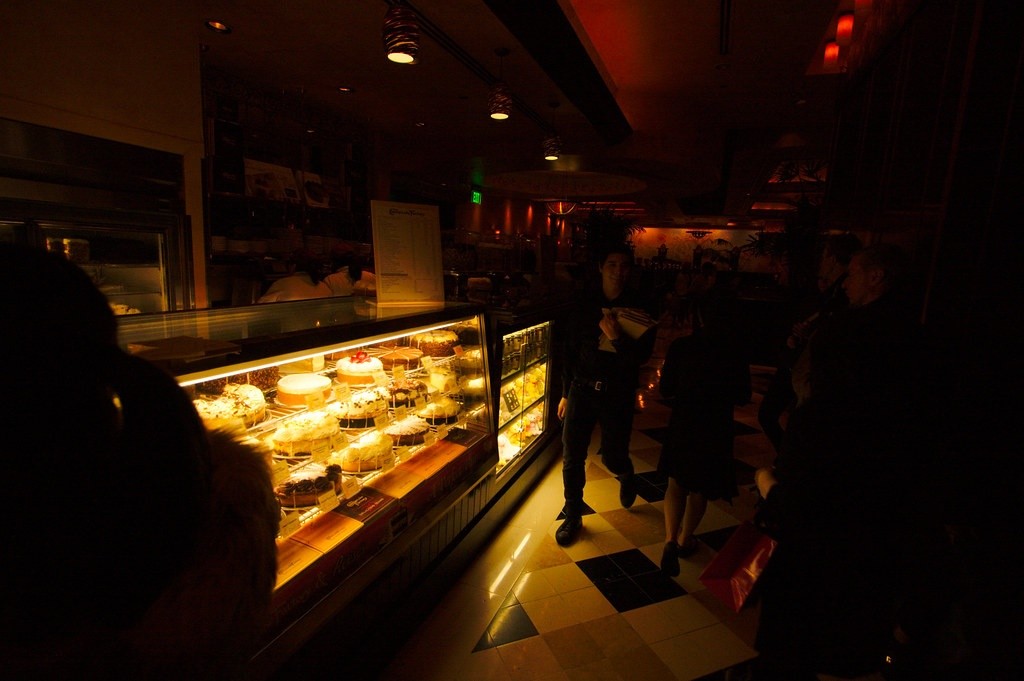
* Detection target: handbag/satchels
[696,505,780,614]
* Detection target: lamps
[382,5,423,66]
[544,100,563,160]
[487,46,513,121]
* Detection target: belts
[594,381,609,393]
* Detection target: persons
[255,247,376,303]
[553,240,657,546]
[657,248,757,577]
[757,236,971,681]
[0,241,278,681]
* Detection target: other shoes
[557,516,582,545]
[616,473,636,509]
[660,540,681,580]
[678,534,698,556]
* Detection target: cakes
[190,323,485,512]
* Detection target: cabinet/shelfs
[112,295,555,681]
